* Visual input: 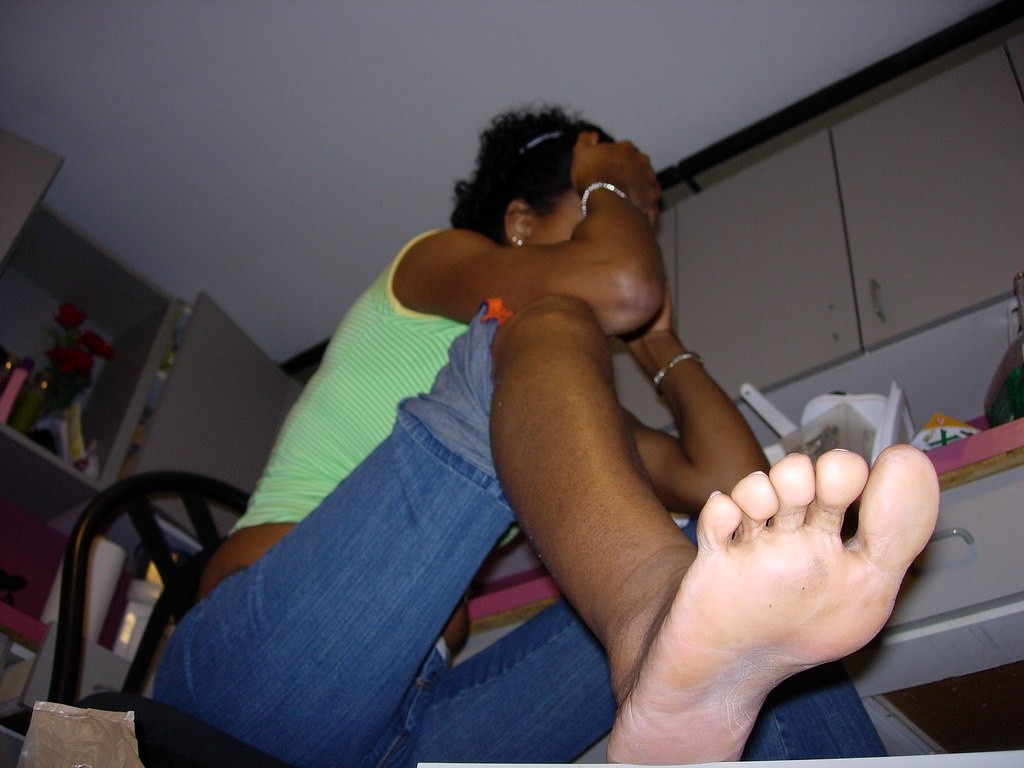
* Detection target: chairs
[50,468,252,712]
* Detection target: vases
[26,373,81,458]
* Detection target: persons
[154,108,939,766]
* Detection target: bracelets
[580,181,627,217]
[653,351,707,388]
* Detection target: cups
[781,402,875,471]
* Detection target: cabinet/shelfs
[594,25,1024,431]
[0,128,309,768]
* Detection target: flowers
[45,304,114,378]
